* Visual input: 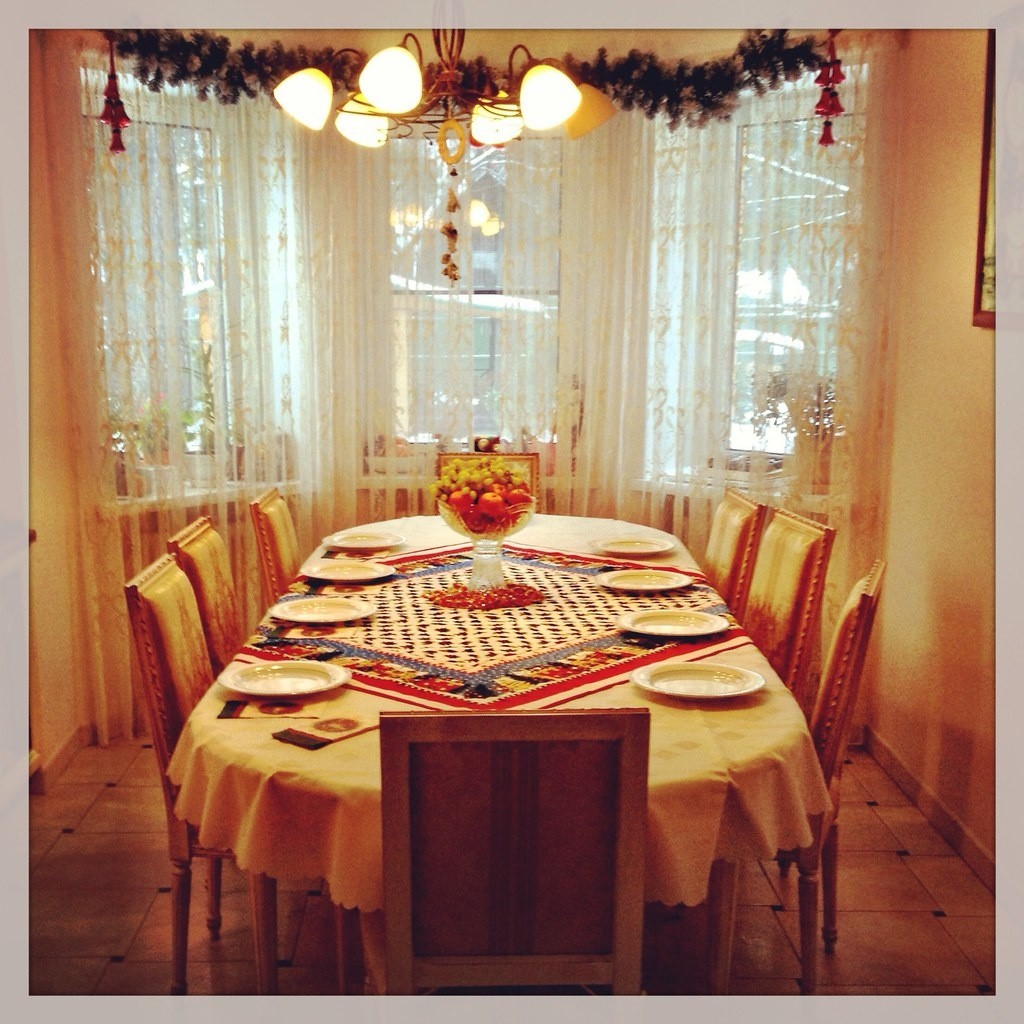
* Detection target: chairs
[167,515,246,679]
[361,708,651,995]
[740,506,837,874]
[435,451,541,514]
[248,489,301,607]
[701,489,769,623]
[124,554,363,995]
[772,558,886,996]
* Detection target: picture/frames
[972,29,996,329]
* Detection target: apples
[448,485,532,527]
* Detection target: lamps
[274,29,617,149]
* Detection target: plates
[320,530,407,550]
[593,570,693,591]
[300,561,396,583]
[266,597,378,623]
[588,536,675,556]
[216,659,352,696]
[628,661,765,698]
[614,610,730,636]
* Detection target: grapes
[430,456,524,500]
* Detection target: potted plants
[116,340,297,495]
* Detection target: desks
[166,515,833,995]
